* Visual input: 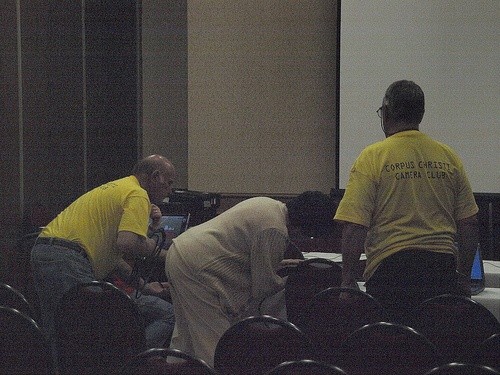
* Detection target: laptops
[453,242,486,282]
[150,212,191,249]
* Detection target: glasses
[376,106,383,118]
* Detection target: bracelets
[148,224,157,233]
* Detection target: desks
[300,250,367,282]
[357,281,500,324]
[456,259,500,288]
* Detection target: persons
[167,191,337,375]
[331,80,479,315]
[107,255,174,351]
[31,154,175,375]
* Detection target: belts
[35,237,87,258]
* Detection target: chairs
[0,231,500,375]
